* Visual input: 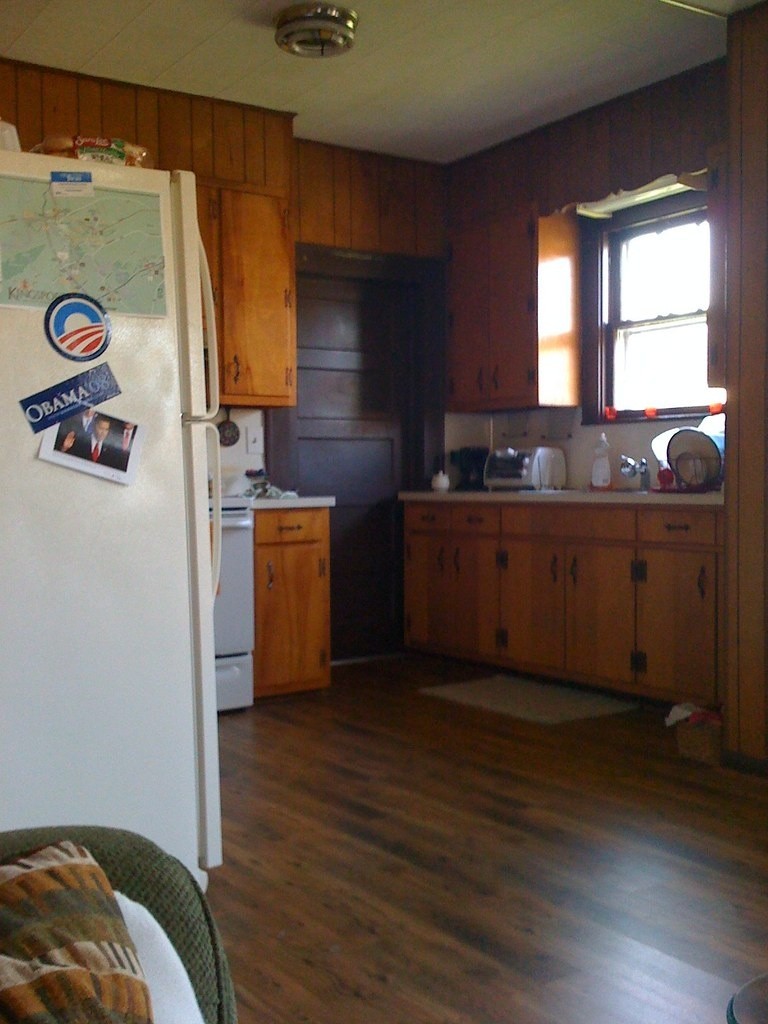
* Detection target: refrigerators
[0,148,221,894]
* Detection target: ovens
[483,446,566,493]
[209,497,255,711]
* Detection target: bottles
[431,470,450,491]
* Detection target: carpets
[417,674,638,725]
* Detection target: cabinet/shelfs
[444,215,582,414]
[253,500,725,713]
[197,176,296,406]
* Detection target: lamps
[274,4,358,60]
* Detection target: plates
[665,428,723,489]
